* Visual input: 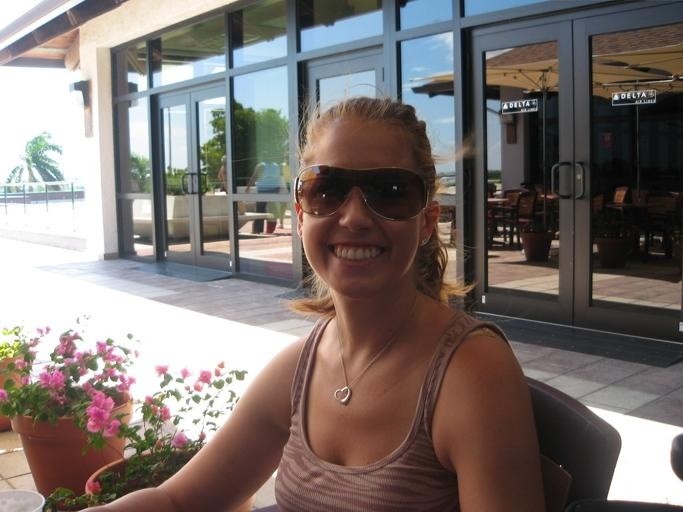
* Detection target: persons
[78,96,548,510]
[276,154,292,229]
[245,150,281,234]
[217,155,245,216]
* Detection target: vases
[10,384,132,512]
[0,353,29,431]
[520,232,551,265]
[85,446,202,500]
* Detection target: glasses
[292,163,430,222]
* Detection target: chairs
[486,186,683,264]
[516,377,622,512]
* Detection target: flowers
[80,361,245,495]
[0,327,48,384]
[0,333,139,445]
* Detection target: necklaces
[332,291,431,403]
